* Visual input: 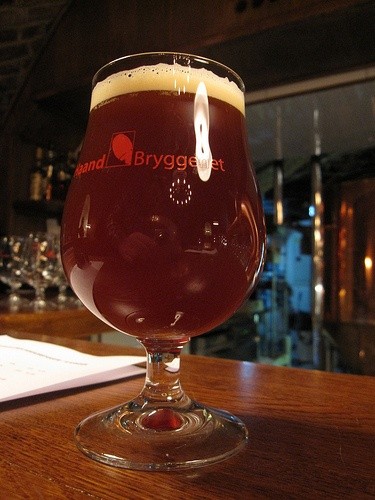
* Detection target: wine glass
[0,234,76,318]
[55,51,265,472]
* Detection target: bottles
[45,146,59,200]
[28,149,45,200]
